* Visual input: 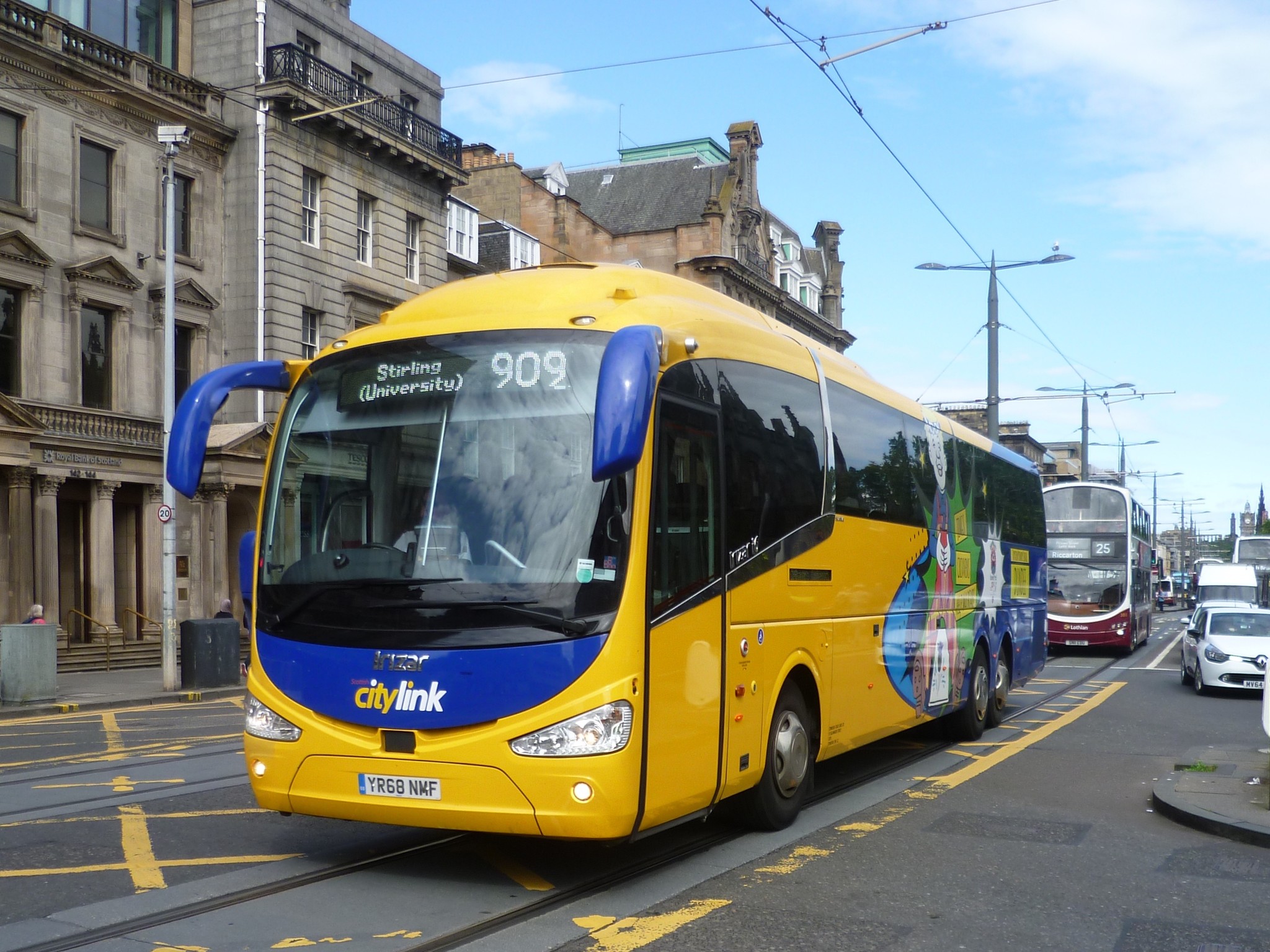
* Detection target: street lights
[914,251,1074,445]
[1135,472,1187,550]
[1159,498,1206,608]
[1089,440,1161,489]
[1035,383,1133,485]
[1173,511,1215,590]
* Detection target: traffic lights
[1151,549,1157,565]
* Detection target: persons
[27,604,46,624]
[394,490,473,569]
[241,612,251,677]
[213,600,233,618]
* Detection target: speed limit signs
[157,505,172,522]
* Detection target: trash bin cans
[180,619,240,689]
[1186,599,1195,608]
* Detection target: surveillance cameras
[158,125,195,144]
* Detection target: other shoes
[241,663,248,677]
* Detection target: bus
[1192,558,1224,603]
[1042,483,1156,654]
[166,260,1050,846]
[1158,576,1178,604]
[1232,536,1270,604]
[1173,573,1191,601]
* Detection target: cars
[1180,600,1256,639]
[1181,607,1270,694]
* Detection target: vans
[1196,564,1260,609]
